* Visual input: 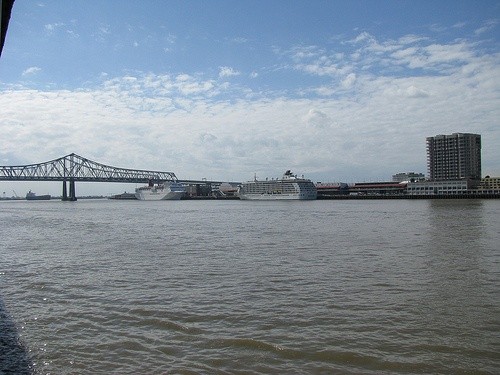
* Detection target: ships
[134,179,187,200]
[238,172,318,200]
